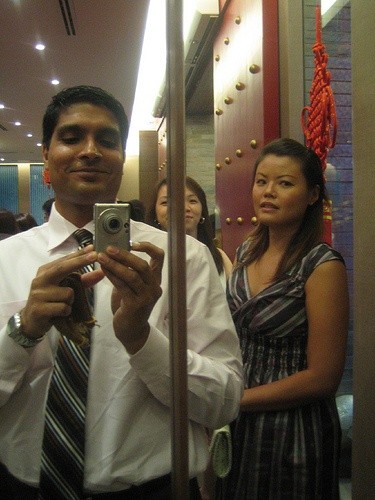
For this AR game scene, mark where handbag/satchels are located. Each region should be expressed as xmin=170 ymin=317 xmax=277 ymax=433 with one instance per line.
xmin=209 ymin=423 xmax=233 ymax=479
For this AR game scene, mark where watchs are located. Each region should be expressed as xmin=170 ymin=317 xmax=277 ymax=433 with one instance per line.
xmin=5 ymin=314 xmax=22 ymax=337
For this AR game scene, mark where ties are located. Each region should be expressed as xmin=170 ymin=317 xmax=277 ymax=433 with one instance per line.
xmin=38 ymin=228 xmax=94 ymax=499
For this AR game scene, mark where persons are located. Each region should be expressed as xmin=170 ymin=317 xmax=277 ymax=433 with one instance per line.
xmin=0 ymin=86 xmax=245 ymax=500
xmin=147 ymin=175 xmax=233 ymax=288
xmin=2 ymin=197 xmax=53 ymax=240
xmin=226 ymin=137 xmax=350 ymax=498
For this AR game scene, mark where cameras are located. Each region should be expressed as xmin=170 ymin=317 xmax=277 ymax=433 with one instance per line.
xmin=92 ymin=202 xmax=131 ymax=259
